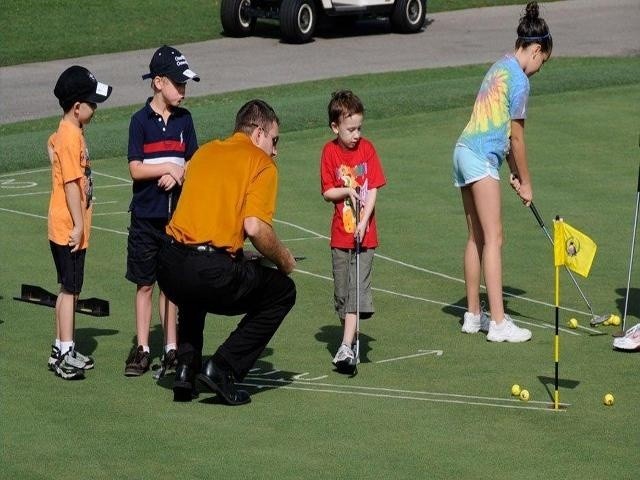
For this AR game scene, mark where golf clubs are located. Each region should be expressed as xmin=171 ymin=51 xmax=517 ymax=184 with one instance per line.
xmin=64 ymin=251 xmax=86 ymax=369
xmin=529 ymin=201 xmax=611 ymax=325
xmin=348 ymin=185 xmax=363 ymax=366
xmin=612 ymin=175 xmax=640 ymax=337
xmin=153 ymin=190 xmax=174 ymax=379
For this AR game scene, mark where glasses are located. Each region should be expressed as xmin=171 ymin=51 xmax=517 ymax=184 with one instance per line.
xmin=251 ymin=122 xmax=279 ymax=146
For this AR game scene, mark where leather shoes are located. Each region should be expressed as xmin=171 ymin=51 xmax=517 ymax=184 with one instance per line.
xmin=198 ymin=358 xmax=252 ymax=405
xmin=172 ymin=365 xmax=199 ymax=399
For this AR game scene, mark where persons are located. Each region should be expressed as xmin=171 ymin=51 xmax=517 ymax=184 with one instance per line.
xmin=453 ymin=0 xmax=553 ymax=344
xmin=46 ymin=66 xmax=114 ymax=379
xmin=157 ymin=99 xmax=297 ymax=406
xmin=124 ymin=43 xmax=200 ymax=375
xmin=320 ymin=89 xmax=387 ymax=374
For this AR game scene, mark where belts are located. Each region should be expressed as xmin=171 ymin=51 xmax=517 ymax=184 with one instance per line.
xmin=168 ymin=239 xmax=220 ymax=253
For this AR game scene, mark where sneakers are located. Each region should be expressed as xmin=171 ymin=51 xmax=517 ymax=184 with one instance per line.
xmin=461 ymin=311 xmax=513 ymax=334
xmin=164 ymin=349 xmax=178 ymax=376
xmin=47 ymin=345 xmax=95 ymax=380
xmin=124 ymin=348 xmax=151 ymax=377
xmin=332 ymin=344 xmax=360 ymax=374
xmin=486 ymin=320 xmax=532 ymax=343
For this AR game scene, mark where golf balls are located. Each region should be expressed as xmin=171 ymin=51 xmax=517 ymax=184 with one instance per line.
xmin=519 ymin=389 xmax=530 ymax=399
xmin=601 ymin=314 xmax=619 ymax=328
xmin=570 ymin=318 xmax=577 ymax=329
xmin=512 ymin=384 xmax=522 ymax=398
xmin=604 ymin=394 xmax=615 ymax=406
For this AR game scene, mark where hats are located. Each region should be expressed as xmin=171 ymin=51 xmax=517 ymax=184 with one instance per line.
xmin=54 ymin=65 xmax=112 ymax=103
xmin=149 ymin=45 xmax=200 ymax=83
